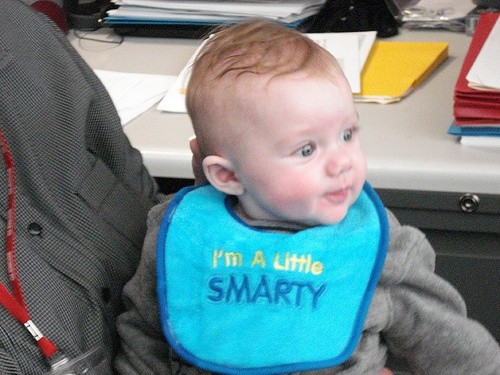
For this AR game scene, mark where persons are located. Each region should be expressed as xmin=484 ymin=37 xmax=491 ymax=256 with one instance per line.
xmin=0 ymin=0 xmax=203 ymax=375
xmin=110 ymin=16 xmax=500 ymax=375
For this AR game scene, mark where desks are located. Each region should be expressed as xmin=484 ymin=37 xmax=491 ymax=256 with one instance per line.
xmin=65 ymin=0 xmax=498 ymax=375
